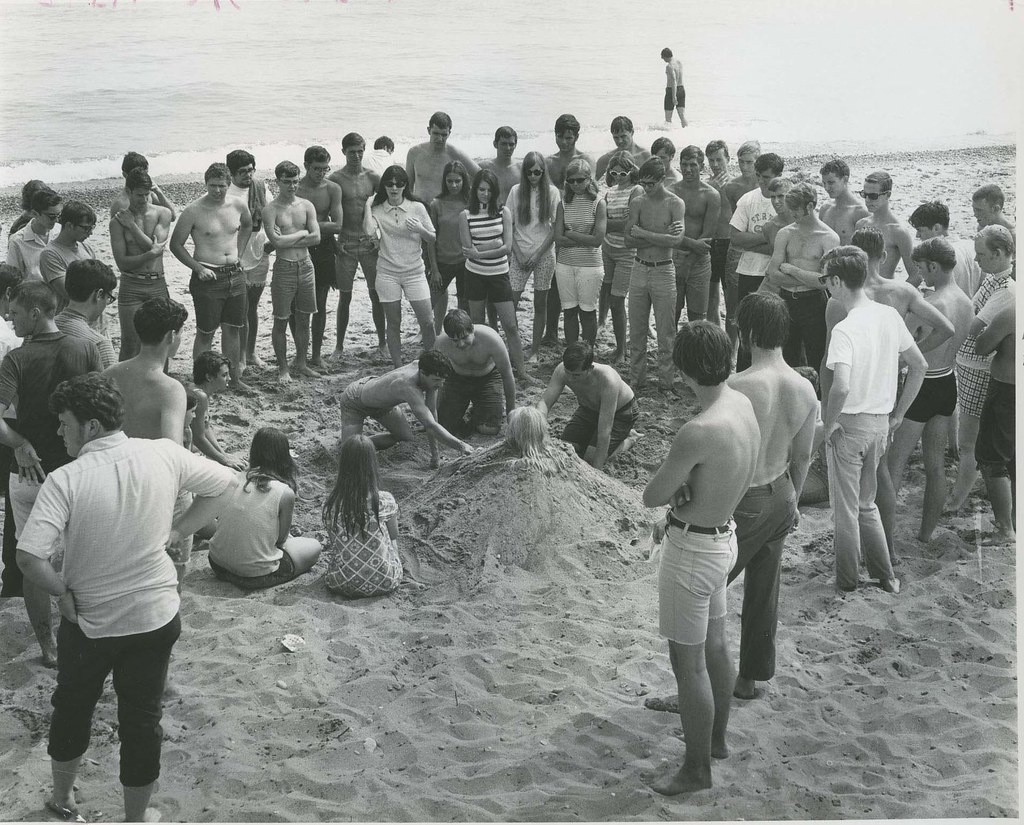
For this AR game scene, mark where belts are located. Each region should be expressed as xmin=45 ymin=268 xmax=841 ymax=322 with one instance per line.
xmin=121 ymin=271 xmax=164 ymax=280
xmin=201 ymin=261 xmax=241 ymax=274
xmin=615 ymin=395 xmax=636 ymax=417
xmin=635 ymin=256 xmax=672 ymax=267
xmin=665 ymin=507 xmax=732 ymax=534
xmin=779 ymin=288 xmax=823 ymax=300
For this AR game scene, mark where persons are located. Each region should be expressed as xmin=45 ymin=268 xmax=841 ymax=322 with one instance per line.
xmin=425 ymin=310 xmax=516 ymax=471
xmin=0 ymin=180 xmax=109 ymax=338
xmin=819 ymin=159 xmax=1016 ymax=593
xmin=111 ymin=151 xmax=176 ymax=222
xmin=0 ymin=262 xmax=24 ymax=598
xmin=208 ymin=427 xmax=320 ymax=589
xmin=661 ymin=47 xmax=688 ymax=128
xmin=170 ymin=112 xmax=840 ymax=400
xmin=0 ymin=281 xmax=104 ymax=670
xmin=643 ymin=320 xmax=760 ymax=795
xmin=101 ymin=298 xmax=244 ymax=663
xmin=110 ymin=168 xmax=172 ymax=374
xmin=787 ymin=366 xmax=830 ymax=506
xmin=16 ymin=371 xmax=239 ymax=822
xmin=644 ymin=290 xmax=818 ymax=714
xmin=322 ymin=434 xmax=403 ymax=597
xmin=536 ymin=341 xmax=643 ymax=472
xmin=506 ymin=406 xmax=565 ymax=472
xmin=54 ymin=258 xmax=118 ymax=369
xmin=340 ymin=350 xmax=474 ymax=470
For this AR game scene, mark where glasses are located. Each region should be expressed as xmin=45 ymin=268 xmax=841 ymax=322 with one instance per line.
xmin=95 ymin=288 xmax=116 ymax=306
xmin=384 ymin=180 xmax=406 ymax=188
xmin=566 ymin=176 xmax=587 ymax=184
xmin=860 ymin=190 xmax=888 ymax=200
xmin=610 ymin=169 xmax=631 ymax=179
xmin=70 ymin=222 xmax=96 ymax=230
xmin=526 ymin=169 xmax=544 ymax=176
xmin=232 ymin=167 xmax=256 ymax=177
xmin=37 ymin=210 xmax=61 ymax=220
xmin=818 ymin=274 xmax=841 ymax=285
xmin=308 ymin=163 xmax=330 ymax=175
